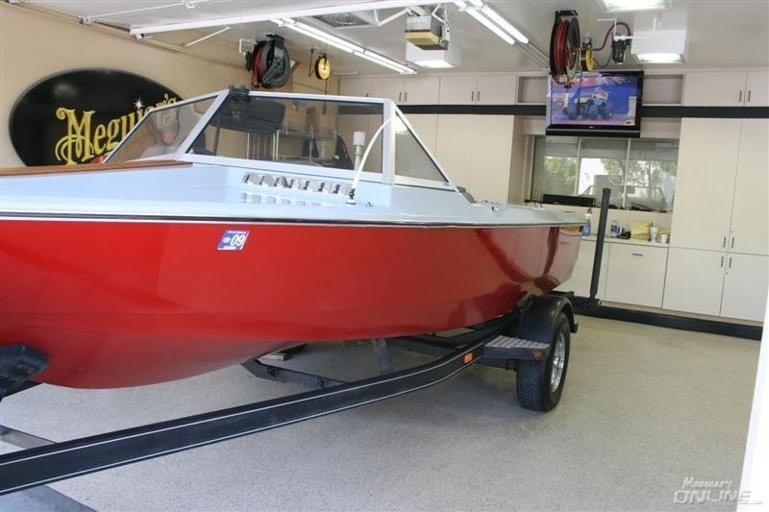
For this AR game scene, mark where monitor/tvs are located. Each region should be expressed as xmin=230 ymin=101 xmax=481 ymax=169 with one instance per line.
xmin=545 ymin=67 xmax=644 ymax=137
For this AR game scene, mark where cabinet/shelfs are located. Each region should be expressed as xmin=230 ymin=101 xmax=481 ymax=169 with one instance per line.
xmin=338 ymin=78 xmax=374 ymax=100
xmin=372 ymin=75 xmax=438 ymax=106
xmin=564 ymin=235 xmax=668 ymax=312
xmin=439 ymin=75 xmax=516 ymax=103
xmin=402 ymin=113 xmax=529 ymax=209
xmin=681 ymin=70 xmax=769 ymax=104
xmin=665 ymin=115 xmax=768 ymax=320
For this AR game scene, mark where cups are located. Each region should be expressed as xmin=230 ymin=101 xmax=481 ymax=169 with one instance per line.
xmin=649 ymin=226 xmax=658 ymax=244
xmin=661 ymin=234 xmax=667 ymax=243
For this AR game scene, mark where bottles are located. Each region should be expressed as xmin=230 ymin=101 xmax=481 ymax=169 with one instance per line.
xmin=610 ymin=218 xmax=619 ymax=238
xmin=583 ymin=219 xmax=591 ymax=236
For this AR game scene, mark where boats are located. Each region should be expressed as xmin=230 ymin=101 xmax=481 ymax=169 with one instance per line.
xmin=0 ymin=80 xmax=590 ymax=395
